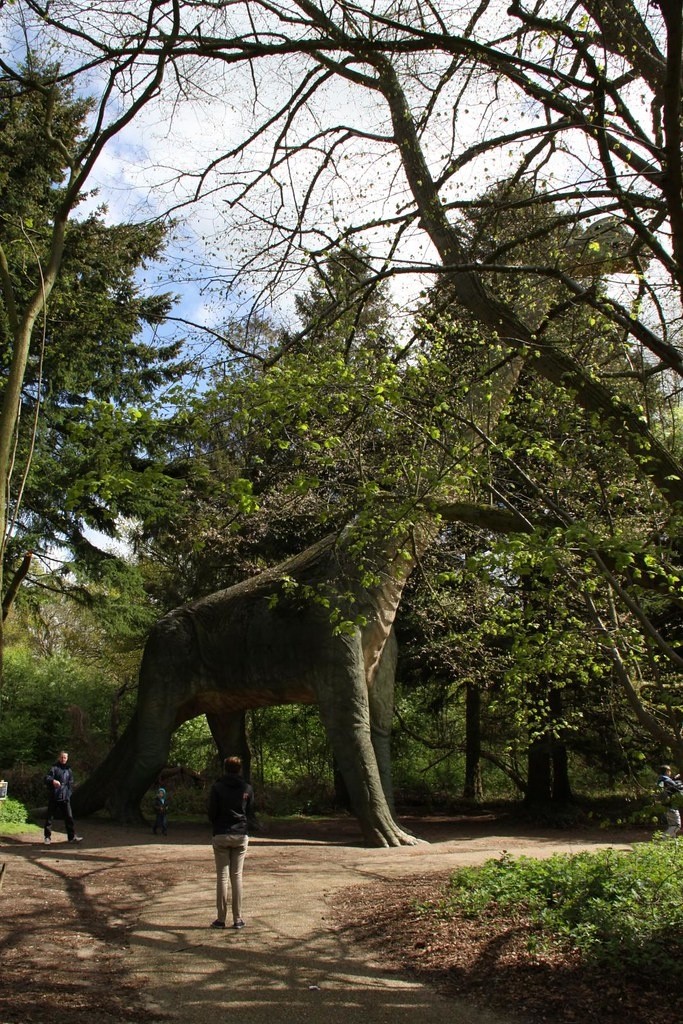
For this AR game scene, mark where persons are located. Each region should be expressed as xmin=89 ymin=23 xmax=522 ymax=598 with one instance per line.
xmin=152 ymin=788 xmax=169 ymax=836
xmin=44 ymin=751 xmax=83 ymax=844
xmin=208 ymin=757 xmax=255 ymax=929
xmin=657 ymin=766 xmax=683 ymax=840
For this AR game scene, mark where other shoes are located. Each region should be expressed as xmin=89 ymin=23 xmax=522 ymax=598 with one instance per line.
xmin=162 ymin=831 xmax=168 ymax=837
xmin=153 ymin=830 xmax=157 ymax=835
xmin=211 ymin=919 xmax=225 ymax=929
xmin=44 ymin=837 xmax=51 ymax=845
xmin=68 ymin=836 xmax=83 ymax=844
xmin=233 ymin=920 xmax=245 ymax=930
